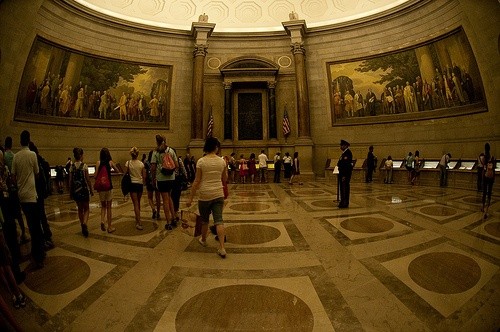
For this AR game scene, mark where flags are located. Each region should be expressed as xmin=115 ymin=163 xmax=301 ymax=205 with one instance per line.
xmin=282 ymin=105 xmax=291 ymax=137
xmin=203 ymin=107 xmax=213 ymax=141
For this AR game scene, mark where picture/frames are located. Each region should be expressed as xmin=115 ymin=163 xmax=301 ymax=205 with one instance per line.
xmin=325 ymin=24 xmax=489 ymax=127
xmin=14 ymin=35 xmax=174 ymax=130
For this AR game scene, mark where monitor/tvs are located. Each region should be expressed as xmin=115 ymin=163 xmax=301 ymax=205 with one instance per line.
xmin=87 ymin=166 xmax=96 ymax=175
xmin=50 ymin=168 xmax=57 ymax=176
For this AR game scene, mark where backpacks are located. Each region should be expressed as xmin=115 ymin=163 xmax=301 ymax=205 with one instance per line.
xmin=94 ymin=165 xmax=110 ymax=190
xmin=70 ymin=164 xmax=87 ymax=198
xmin=160 ymin=146 xmax=175 ymax=175
xmin=386 ymin=161 xmax=391 ymax=169
xmin=484 ymin=158 xmax=495 ymax=178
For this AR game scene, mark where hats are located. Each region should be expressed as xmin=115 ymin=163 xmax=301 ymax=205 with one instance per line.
xmin=340 ymin=139 xmax=350 ymax=146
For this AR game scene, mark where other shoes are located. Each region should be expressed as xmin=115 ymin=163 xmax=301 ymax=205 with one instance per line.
xmin=107 ymin=227 xmax=115 ymax=233
xmin=14 ymin=251 xmax=31 ymax=260
xmin=136 ymin=224 xmax=143 ymax=230
xmin=158 ymin=214 xmax=161 ymax=217
xmin=20 ymin=235 xmax=30 ymax=240
xmin=44 ymin=240 xmax=54 ymax=250
xmin=164 ymin=224 xmax=172 ymax=230
xmin=152 ymin=210 xmax=157 ymax=219
xmin=171 ymin=219 xmax=176 ymax=227
xmin=215 ymin=235 xmax=226 ymax=242
xmin=82 ymin=223 xmax=89 ymax=237
xmin=411 ymin=181 xmax=414 ymax=184
xmin=484 ymin=214 xmax=488 ymax=218
xmin=217 ymin=248 xmax=226 ymax=257
xmin=101 ymin=222 xmax=106 ymax=231
xmin=232 ymin=180 xmax=303 ymax=185
xmin=199 ymin=237 xmax=207 ymax=246
xmin=338 ymin=205 xmax=350 ymax=208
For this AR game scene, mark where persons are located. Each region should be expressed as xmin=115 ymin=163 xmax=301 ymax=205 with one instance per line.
xmin=222 ymin=150 xmax=305 ymax=184
xmin=337 ymin=138 xmax=353 ymax=209
xmin=69 ymin=147 xmax=93 ymax=238
xmin=142 ymin=135 xmax=197 ymax=229
xmin=365 ymin=145 xmax=452 ymax=186
xmin=0 ymin=130 xmax=74 ymax=309
xmin=93 ymin=148 xmax=119 ymax=233
xmin=334 ymin=63 xmax=477 ymax=120
xmin=123 ymin=146 xmax=146 ymax=230
xmin=205 ymin=150 xmax=229 ymax=242
xmin=187 ymin=137 xmax=229 ymax=256
xmin=26 ymin=72 xmax=166 ymax=122
xmin=475 ymin=143 xmax=497 ymax=218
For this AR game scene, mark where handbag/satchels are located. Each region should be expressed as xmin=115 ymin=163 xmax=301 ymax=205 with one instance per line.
xmin=181 ymin=204 xmax=201 ymax=235
xmin=243 ymin=164 xmax=249 ymax=170
xmin=178 ymin=174 xmax=188 ymax=191
xmin=229 ymin=164 xmax=234 ymax=170
xmin=121 ymin=162 xmax=132 ymax=196
